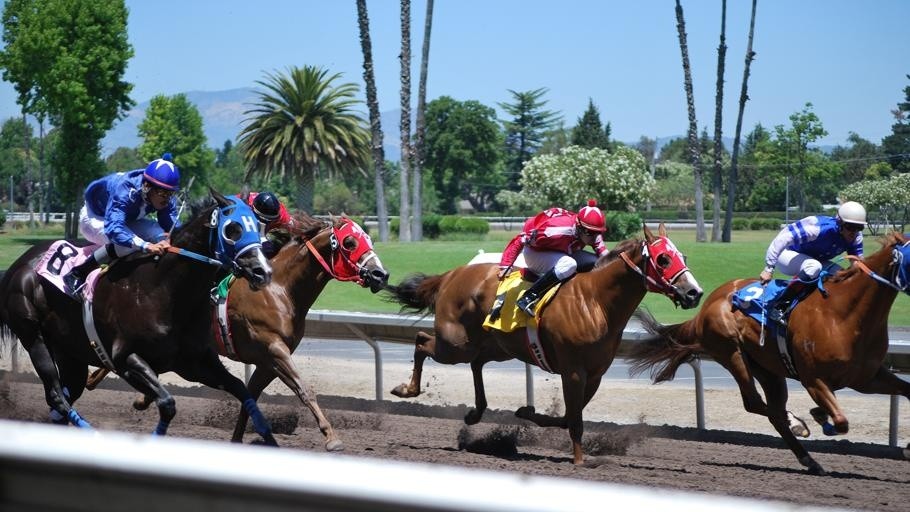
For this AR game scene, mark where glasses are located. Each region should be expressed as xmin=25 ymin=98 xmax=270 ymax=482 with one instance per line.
xmin=584 ymin=232 xmax=602 ymax=238
xmin=157 ymin=190 xmax=174 ymax=199
xmin=844 ymin=223 xmax=864 ymax=232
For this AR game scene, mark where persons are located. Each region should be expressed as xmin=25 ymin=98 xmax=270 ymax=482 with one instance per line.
xmin=209 ymin=190 xmax=305 ymax=307
xmin=757 ymin=199 xmax=870 ymax=328
xmin=59 ymin=157 xmax=186 ymax=304
xmin=495 ymin=204 xmax=610 ymax=319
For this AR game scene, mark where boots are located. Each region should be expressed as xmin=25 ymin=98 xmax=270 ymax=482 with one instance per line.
xmin=768 ymin=270 xmax=813 ymax=326
xmin=516 ymin=267 xmax=559 ymax=318
xmin=63 ymin=243 xmax=118 ymax=303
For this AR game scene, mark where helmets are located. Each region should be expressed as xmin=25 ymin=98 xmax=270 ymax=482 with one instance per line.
xmin=252 ymin=192 xmax=282 ymax=221
xmin=142 ymin=152 xmax=182 ymax=191
xmin=836 ymin=200 xmax=868 ymax=225
xmin=577 ymin=200 xmax=607 ymax=232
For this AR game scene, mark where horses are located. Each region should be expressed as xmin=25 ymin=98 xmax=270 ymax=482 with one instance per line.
xmin=132 ymin=209 xmax=391 ymax=452
xmin=375 ymin=220 xmax=705 ymax=467
xmin=620 ymin=229 xmax=910 ymax=476
xmin=0 ymin=181 xmax=280 ymax=448
